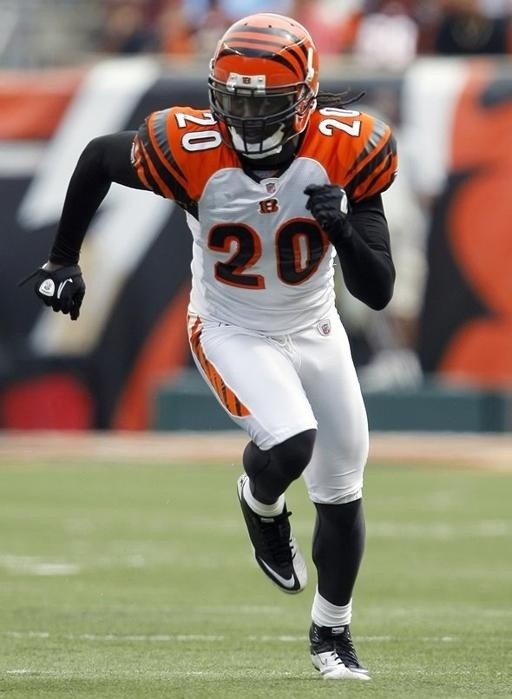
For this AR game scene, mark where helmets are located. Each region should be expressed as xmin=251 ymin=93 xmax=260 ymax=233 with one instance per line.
xmin=208 ymin=12 xmax=320 ymax=166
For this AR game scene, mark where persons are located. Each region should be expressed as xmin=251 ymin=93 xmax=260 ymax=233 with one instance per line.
xmin=31 ymin=11 xmax=398 ymax=684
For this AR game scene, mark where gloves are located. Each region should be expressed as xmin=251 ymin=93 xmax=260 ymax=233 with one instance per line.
xmin=303 ymin=182 xmax=350 ymax=231
xmin=32 ymin=263 xmax=86 ymax=319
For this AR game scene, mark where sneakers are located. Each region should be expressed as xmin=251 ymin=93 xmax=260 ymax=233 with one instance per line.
xmin=309 ymin=622 xmax=372 ymax=682
xmin=236 ymin=472 xmax=310 ymax=594
